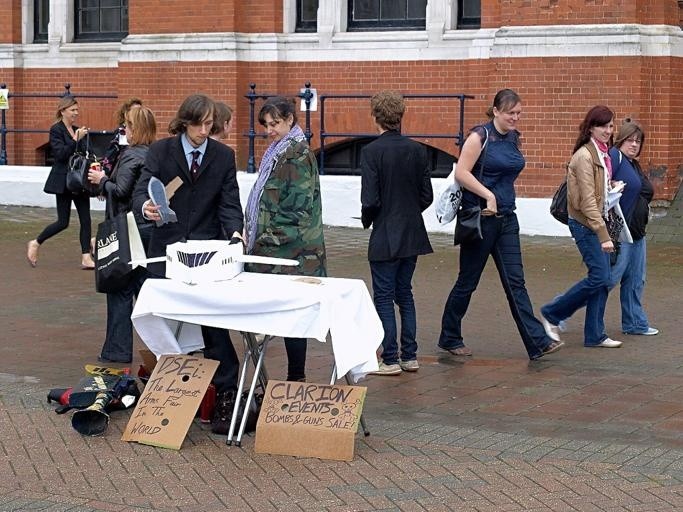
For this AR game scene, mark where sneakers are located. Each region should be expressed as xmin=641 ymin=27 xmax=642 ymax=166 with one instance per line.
xmin=540 ymin=306 xmax=561 ymax=342
xmin=367 ymin=359 xmax=402 ymax=376
xmin=623 ymin=327 xmax=659 ymax=336
xmin=401 ymin=357 xmax=420 ymax=371
xmin=595 ymin=336 xmax=622 ymax=349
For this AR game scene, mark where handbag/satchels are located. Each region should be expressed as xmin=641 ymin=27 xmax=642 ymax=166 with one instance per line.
xmin=94 ymin=179 xmax=148 ymax=293
xmin=65 ymin=125 xmax=101 ymax=200
xmin=451 ymin=125 xmax=483 ymax=248
xmin=437 ymin=161 xmax=463 ymax=226
xmin=549 ymin=160 xmax=571 ymax=227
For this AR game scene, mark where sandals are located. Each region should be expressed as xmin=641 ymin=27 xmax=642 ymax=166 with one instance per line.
xmin=26 ymin=240 xmax=38 ymax=267
xmin=80 ymin=259 xmax=96 ymax=270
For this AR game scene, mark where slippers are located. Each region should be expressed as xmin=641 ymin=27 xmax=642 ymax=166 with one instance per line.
xmin=437 ymin=342 xmax=473 ymax=357
xmin=529 ymin=341 xmax=565 ymax=362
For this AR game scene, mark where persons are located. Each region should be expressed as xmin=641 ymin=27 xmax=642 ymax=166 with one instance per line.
xmin=549 ymin=122 xmax=659 ymax=336
xmin=434 ymin=88 xmax=564 ymax=361
xmin=25 ymin=92 xmax=99 ymax=270
xmin=132 ymin=94 xmax=244 ymax=388
xmin=245 ymin=96 xmax=327 ymax=387
xmin=538 ymin=104 xmax=633 ymax=349
xmin=88 ymin=107 xmax=157 ymax=363
xmin=209 ymin=102 xmax=233 ymax=142
xmin=101 ymin=97 xmax=143 ymax=177
xmin=360 ymin=89 xmax=434 ymax=376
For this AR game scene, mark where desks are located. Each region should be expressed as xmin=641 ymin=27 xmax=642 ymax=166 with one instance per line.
xmin=130 ymin=275 xmax=386 ymax=448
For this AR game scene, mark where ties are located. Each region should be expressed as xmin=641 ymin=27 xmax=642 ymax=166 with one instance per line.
xmin=189 ymin=150 xmax=201 ymax=184
